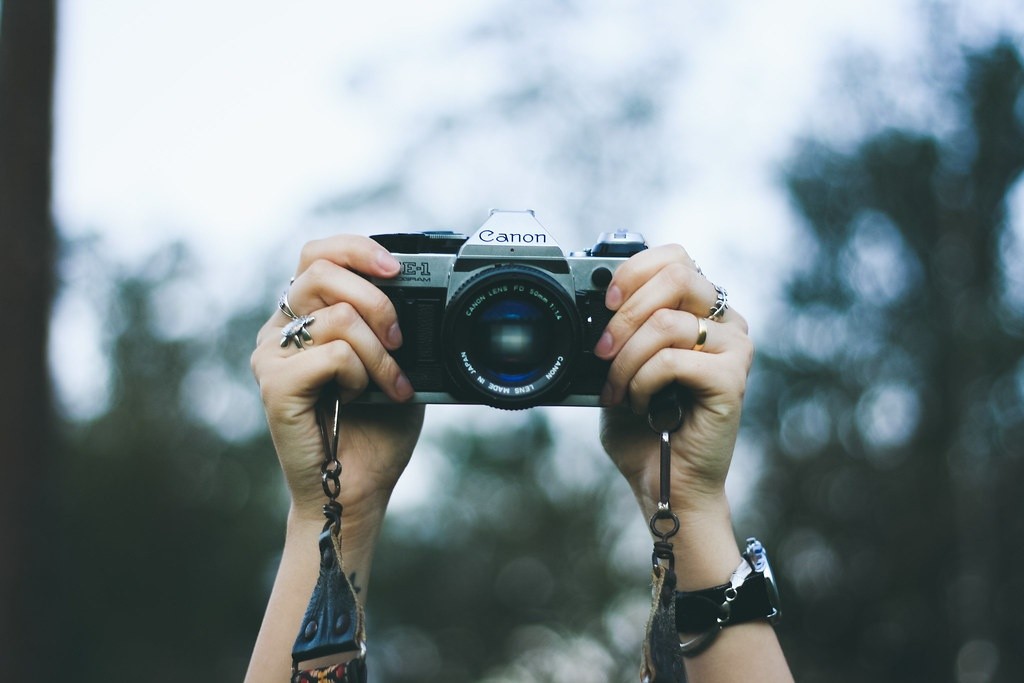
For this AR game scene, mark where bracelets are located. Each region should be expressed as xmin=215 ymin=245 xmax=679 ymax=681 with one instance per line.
xmin=678 ymin=557 xmax=755 ymax=657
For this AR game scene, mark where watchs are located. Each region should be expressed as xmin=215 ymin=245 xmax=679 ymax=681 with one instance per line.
xmin=676 ymin=536 xmax=784 ymax=631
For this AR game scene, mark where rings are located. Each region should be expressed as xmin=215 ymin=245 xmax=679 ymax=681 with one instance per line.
xmin=707 ymin=282 xmax=731 ymax=321
xmin=277 ymin=288 xmax=298 ymax=320
xmin=692 ymin=313 xmax=706 ymax=351
xmin=693 ymin=259 xmax=707 ymax=277
xmin=288 ymin=275 xmax=296 ymax=285
xmin=280 ymin=312 xmax=315 ymax=351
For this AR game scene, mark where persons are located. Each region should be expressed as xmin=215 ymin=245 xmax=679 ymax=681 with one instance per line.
xmin=245 ymin=235 xmax=795 ymax=683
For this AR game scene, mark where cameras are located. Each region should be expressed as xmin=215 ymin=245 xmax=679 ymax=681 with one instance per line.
xmin=330 ymin=208 xmax=649 ymax=409
